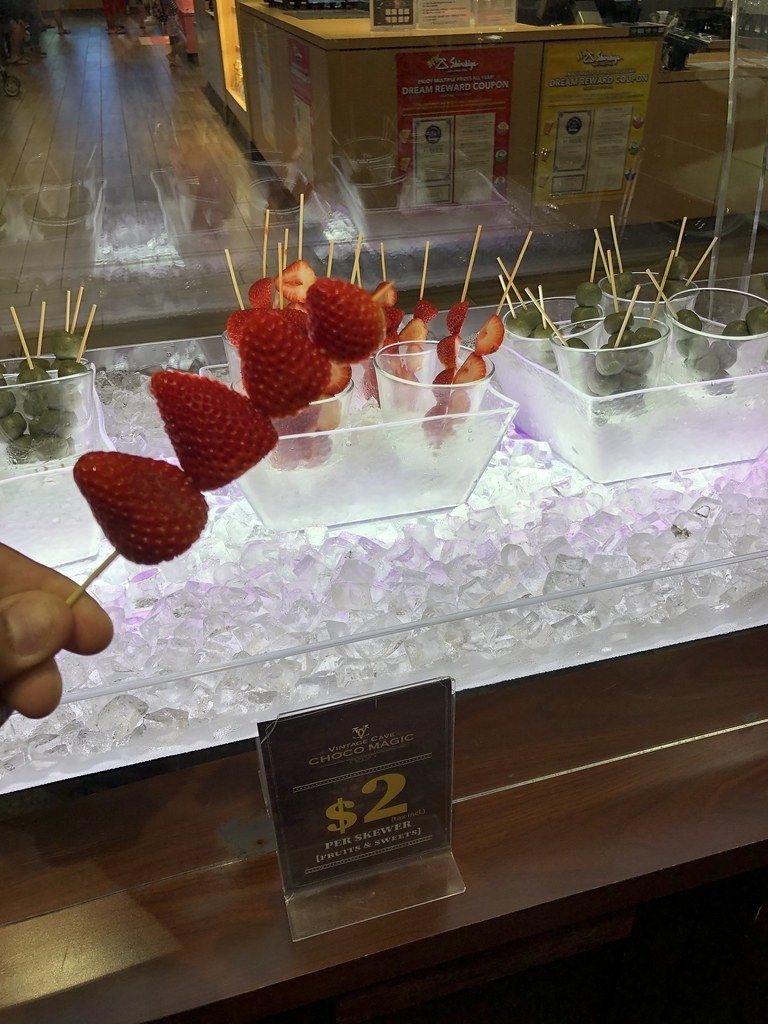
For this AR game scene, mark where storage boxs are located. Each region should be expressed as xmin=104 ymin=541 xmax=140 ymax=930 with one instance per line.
xmin=197 ymin=363 xmax=521 ymax=532
xmin=1 ymin=390 xmax=122 ymax=569
xmin=493 ymin=332 xmax=768 ymax=485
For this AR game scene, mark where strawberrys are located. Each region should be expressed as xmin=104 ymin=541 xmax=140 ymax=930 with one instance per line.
xmin=71 ymin=258 xmax=505 ymax=564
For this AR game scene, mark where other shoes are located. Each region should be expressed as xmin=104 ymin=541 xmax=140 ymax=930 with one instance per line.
xmin=58 ymin=29 xmax=71 ymax=34
xmin=106 ymin=25 xmax=126 ymax=34
xmin=43 ymin=23 xmax=55 ymax=28
xmin=166 ymin=54 xmax=179 ymax=67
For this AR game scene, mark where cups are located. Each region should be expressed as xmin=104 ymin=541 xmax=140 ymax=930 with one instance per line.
xmin=501 ymin=271 xmax=768 ymax=399
xmin=222 ymin=320 xmax=497 ymax=473
xmin=0 ymin=356 xmax=96 ymax=472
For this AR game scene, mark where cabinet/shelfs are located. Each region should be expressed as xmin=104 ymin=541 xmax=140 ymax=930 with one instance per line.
xmin=192 ymin=0 xmax=663 ymax=236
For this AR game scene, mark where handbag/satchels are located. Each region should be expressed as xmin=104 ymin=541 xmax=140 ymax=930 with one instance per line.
xmin=165 ymin=13 xmax=184 ymax=37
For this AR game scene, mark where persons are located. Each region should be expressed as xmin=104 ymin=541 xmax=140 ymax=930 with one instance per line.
xmin=0 ymin=0 xmax=208 ymax=72
xmin=0 ymin=547 xmax=117 ymax=741
